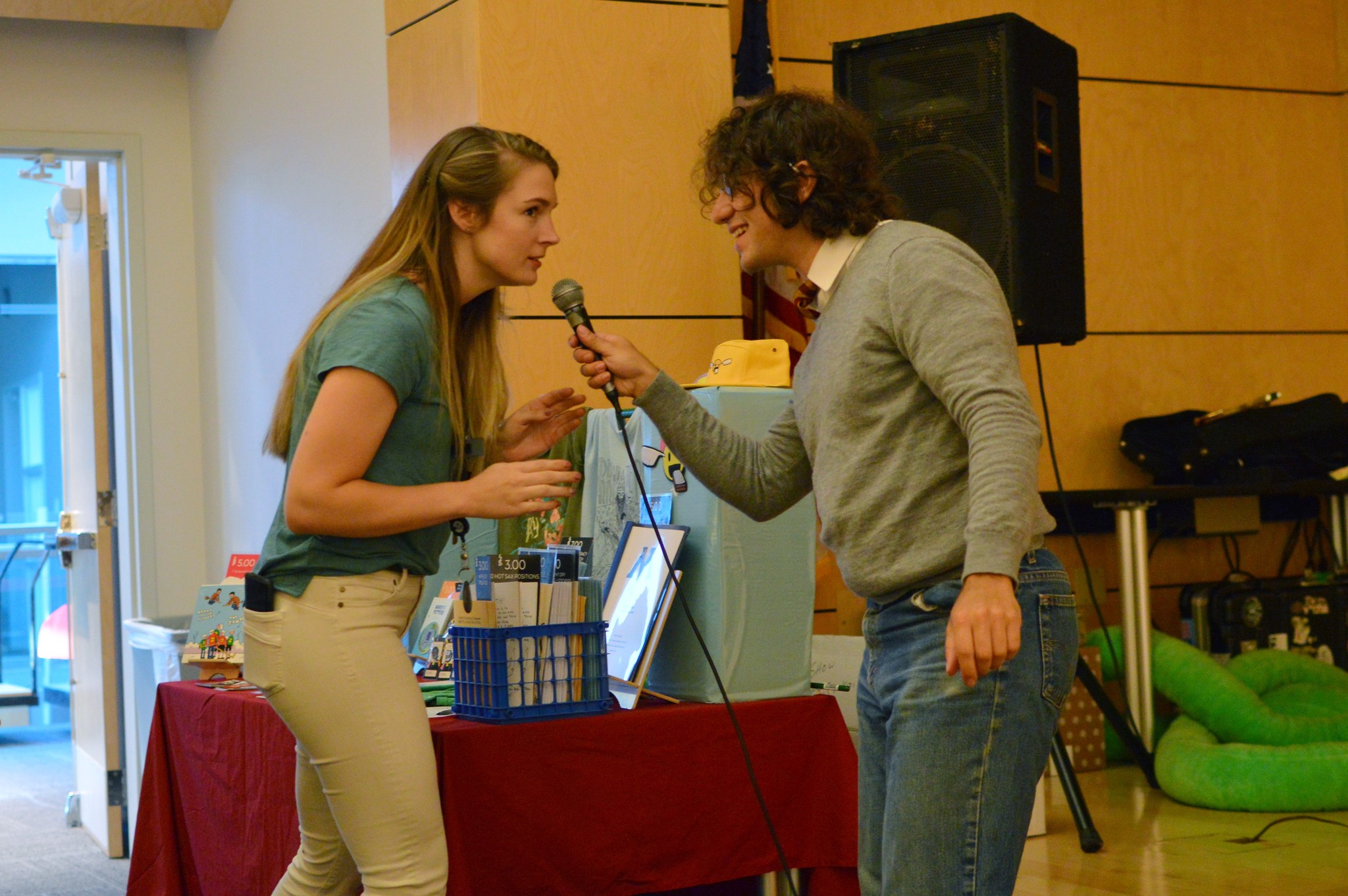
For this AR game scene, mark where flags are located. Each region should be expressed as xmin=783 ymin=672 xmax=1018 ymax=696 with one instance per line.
xmin=733 ymin=0 xmax=822 ymax=376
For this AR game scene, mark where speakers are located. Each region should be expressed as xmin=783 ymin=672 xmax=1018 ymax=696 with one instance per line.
xmin=831 ymin=13 xmax=1088 ymax=348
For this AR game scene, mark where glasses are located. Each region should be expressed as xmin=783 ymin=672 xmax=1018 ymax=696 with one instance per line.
xmin=716 ymin=169 xmax=767 ymax=197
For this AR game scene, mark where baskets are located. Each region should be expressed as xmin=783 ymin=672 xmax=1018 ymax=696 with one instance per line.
xmin=447 ymin=621 xmax=613 ymax=724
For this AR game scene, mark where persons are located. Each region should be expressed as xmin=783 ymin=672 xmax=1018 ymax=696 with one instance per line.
xmin=240 ymin=125 xmax=588 ymax=895
xmin=568 ymin=87 xmax=1081 ymax=896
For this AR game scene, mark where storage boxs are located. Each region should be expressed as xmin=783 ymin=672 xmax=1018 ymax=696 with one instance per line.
xmin=448 ymin=620 xmax=614 ymax=725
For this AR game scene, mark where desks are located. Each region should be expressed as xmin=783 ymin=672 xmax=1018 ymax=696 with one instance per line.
xmin=125 ymin=674 xmax=863 ymax=895
xmin=1037 ymin=486 xmax=1348 ymax=758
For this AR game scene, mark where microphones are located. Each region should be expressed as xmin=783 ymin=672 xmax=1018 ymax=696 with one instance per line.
xmin=550 ymin=278 xmax=623 ymax=414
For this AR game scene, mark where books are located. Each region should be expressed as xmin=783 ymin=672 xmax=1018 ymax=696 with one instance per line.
xmin=410 ymin=580 xmax=463 ymax=657
xmin=181 ymin=554 xmax=261 ymax=668
